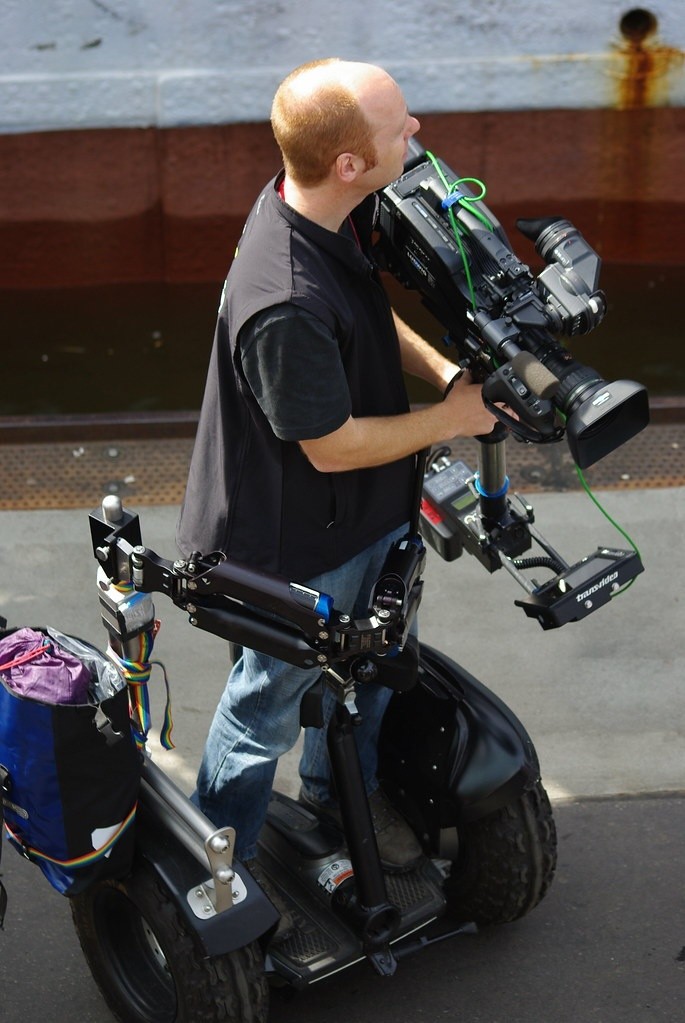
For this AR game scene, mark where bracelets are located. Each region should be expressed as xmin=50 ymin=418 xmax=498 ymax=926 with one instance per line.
xmin=443 ymin=367 xmax=469 ymax=399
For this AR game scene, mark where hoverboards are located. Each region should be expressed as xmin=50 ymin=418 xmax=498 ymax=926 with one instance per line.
xmin=1 ymin=481 xmax=563 ymax=1023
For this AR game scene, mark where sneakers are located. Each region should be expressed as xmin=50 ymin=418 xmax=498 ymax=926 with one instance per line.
xmin=298 ymin=779 xmax=426 ymax=876
xmin=237 ymin=854 xmax=297 ymax=946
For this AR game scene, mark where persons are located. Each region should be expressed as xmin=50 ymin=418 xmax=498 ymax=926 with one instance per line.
xmin=176 ymin=58 xmax=519 ymax=944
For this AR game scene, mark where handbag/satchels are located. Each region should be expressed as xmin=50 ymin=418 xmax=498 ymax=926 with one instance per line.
xmin=0 ymin=627 xmax=145 ymax=896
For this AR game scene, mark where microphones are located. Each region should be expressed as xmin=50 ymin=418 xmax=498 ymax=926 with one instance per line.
xmin=473 ymin=310 xmax=560 ymax=401
